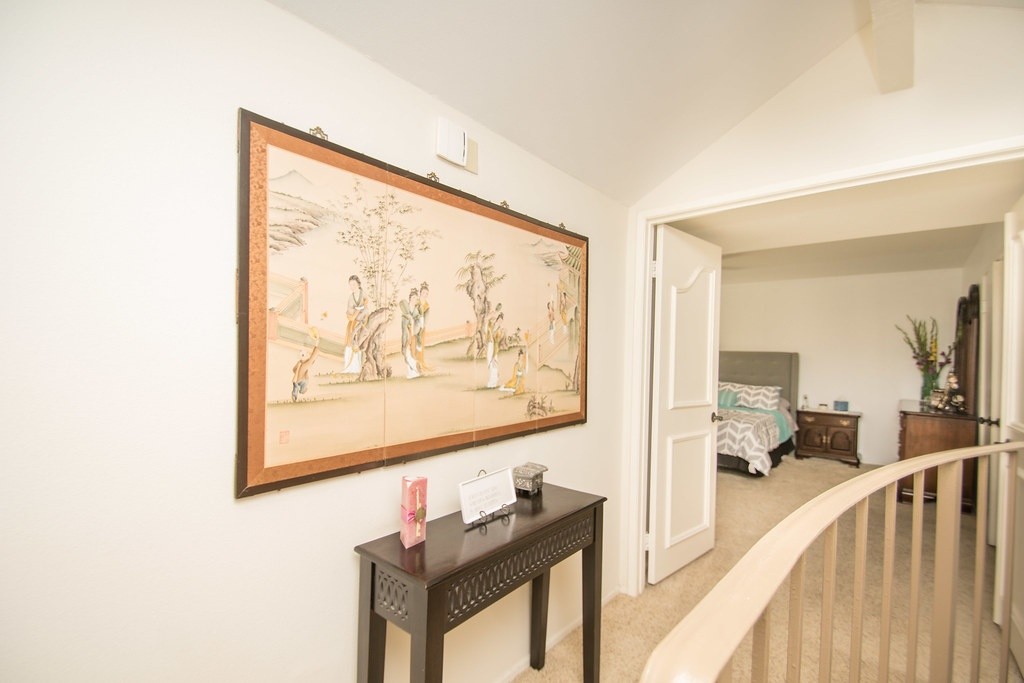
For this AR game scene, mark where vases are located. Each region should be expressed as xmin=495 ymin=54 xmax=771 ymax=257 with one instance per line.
xmin=922 ymin=374 xmax=939 ymax=398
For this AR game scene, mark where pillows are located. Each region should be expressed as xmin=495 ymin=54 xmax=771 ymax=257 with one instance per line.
xmin=718 ymin=381 xmax=790 ymax=411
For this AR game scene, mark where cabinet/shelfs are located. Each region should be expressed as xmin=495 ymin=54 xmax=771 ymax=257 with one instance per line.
xmin=897 ymin=399 xmax=978 ymax=513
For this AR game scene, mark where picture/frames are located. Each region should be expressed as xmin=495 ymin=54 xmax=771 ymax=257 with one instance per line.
xmin=236 ymin=108 xmax=589 ymax=501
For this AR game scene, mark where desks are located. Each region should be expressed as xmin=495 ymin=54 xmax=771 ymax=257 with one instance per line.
xmin=354 ymin=482 xmax=608 ymax=683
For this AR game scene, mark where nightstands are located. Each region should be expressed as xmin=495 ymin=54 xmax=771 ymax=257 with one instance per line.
xmin=796 ymin=410 xmax=863 ymax=468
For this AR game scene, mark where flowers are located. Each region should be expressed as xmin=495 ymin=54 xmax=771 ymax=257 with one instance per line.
xmin=895 ymin=314 xmax=958 ymax=380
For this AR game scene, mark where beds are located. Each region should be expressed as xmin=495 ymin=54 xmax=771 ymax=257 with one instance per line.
xmin=717 ymin=350 xmax=799 ymax=477
